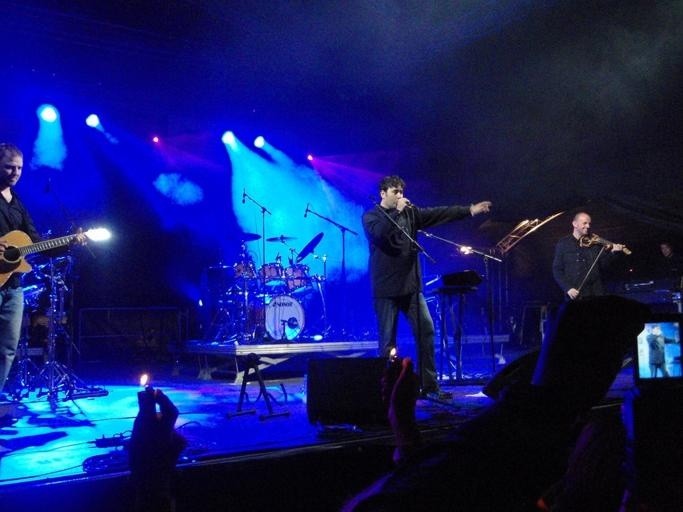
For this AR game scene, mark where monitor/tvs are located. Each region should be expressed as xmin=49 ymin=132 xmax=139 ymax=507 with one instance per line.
xmin=633 ymin=312 xmax=683 ymax=389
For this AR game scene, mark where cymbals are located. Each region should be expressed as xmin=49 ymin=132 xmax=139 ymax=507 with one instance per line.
xmin=267 ymin=237 xmax=292 ymax=241
xmin=226 ymin=233 xmax=261 ymax=241
xmin=209 ymin=266 xmax=231 ymax=270
xmin=296 ymin=232 xmax=324 ymax=262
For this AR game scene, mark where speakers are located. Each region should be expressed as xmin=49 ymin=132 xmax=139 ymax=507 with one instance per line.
xmin=307 ymin=357 xmax=404 ymax=425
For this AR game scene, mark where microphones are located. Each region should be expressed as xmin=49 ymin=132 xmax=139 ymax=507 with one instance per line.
xmin=242 ymin=187 xmax=245 ymax=204
xmin=406 ymin=202 xmax=413 ymax=209
xmin=369 ymin=195 xmax=377 ymax=206
xmin=304 ymin=203 xmax=309 ymax=218
xmin=43 ymin=177 xmax=52 ymax=192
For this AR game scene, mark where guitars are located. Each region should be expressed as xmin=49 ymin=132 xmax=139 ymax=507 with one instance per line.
xmin=0 ymin=228 xmax=112 ymax=287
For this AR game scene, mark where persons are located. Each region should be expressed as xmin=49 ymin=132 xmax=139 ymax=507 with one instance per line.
xmin=550 ymin=212 xmax=626 ymax=303
xmin=359 ymin=174 xmax=493 ymax=400
xmin=342 ymin=294 xmax=656 ymax=512
xmin=0 ymin=141 xmax=90 ymax=394
xmin=657 ymin=239 xmax=682 ymax=277
xmin=377 ymin=354 xmax=425 ymax=467
xmin=125 ymin=384 xmax=189 ymax=511
xmin=644 ymin=322 xmax=681 ymax=379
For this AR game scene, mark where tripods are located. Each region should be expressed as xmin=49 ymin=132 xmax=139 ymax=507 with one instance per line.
xmin=204 ymin=213 xmax=358 ymax=342
xmin=375 ymin=207 xmax=462 ymax=412
xmin=11 ymin=255 xmax=89 ymax=414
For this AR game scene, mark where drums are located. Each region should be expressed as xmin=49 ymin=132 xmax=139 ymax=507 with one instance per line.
xmin=232 ymin=263 xmax=256 ymax=281
xmin=258 ymin=262 xmax=286 ymax=286
xmin=256 ymin=295 xmax=304 ymax=339
xmin=284 ymin=265 xmax=313 ymax=295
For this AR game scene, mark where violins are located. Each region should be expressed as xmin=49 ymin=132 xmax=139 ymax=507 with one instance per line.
xmin=580 ymin=232 xmax=631 ymax=256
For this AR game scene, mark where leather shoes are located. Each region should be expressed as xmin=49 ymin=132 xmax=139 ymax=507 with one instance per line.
xmin=422 ymin=388 xmax=452 ymax=398
